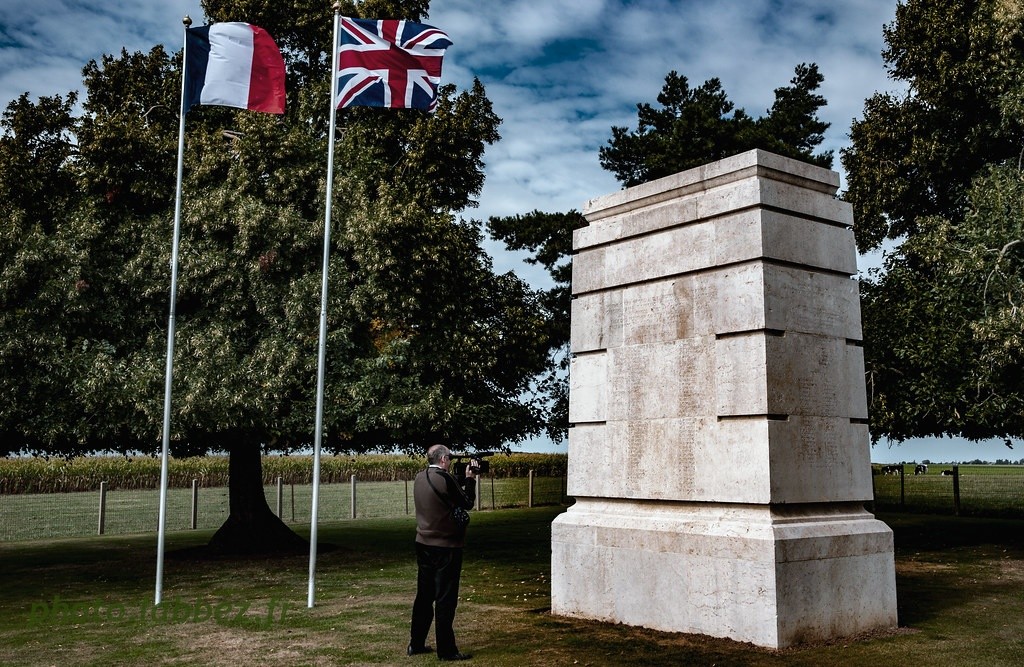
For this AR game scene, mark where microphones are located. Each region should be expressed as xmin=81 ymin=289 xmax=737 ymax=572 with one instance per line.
xmin=474 ymin=452 xmax=494 ymax=458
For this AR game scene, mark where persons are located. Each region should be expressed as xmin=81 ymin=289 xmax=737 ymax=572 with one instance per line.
xmin=407 ymin=445 xmax=481 ymax=661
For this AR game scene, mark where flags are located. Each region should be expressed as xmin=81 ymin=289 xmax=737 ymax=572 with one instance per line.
xmin=183 ymin=22 xmax=285 ymax=116
xmin=334 ymin=15 xmax=454 ymax=110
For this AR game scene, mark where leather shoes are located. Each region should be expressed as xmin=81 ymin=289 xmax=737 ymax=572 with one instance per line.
xmin=408 ymin=645 xmax=434 ymax=655
xmin=439 ymin=653 xmax=472 ymax=661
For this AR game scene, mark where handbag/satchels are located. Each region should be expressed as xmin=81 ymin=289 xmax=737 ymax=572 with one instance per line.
xmin=446 ymin=504 xmax=470 ymax=528
